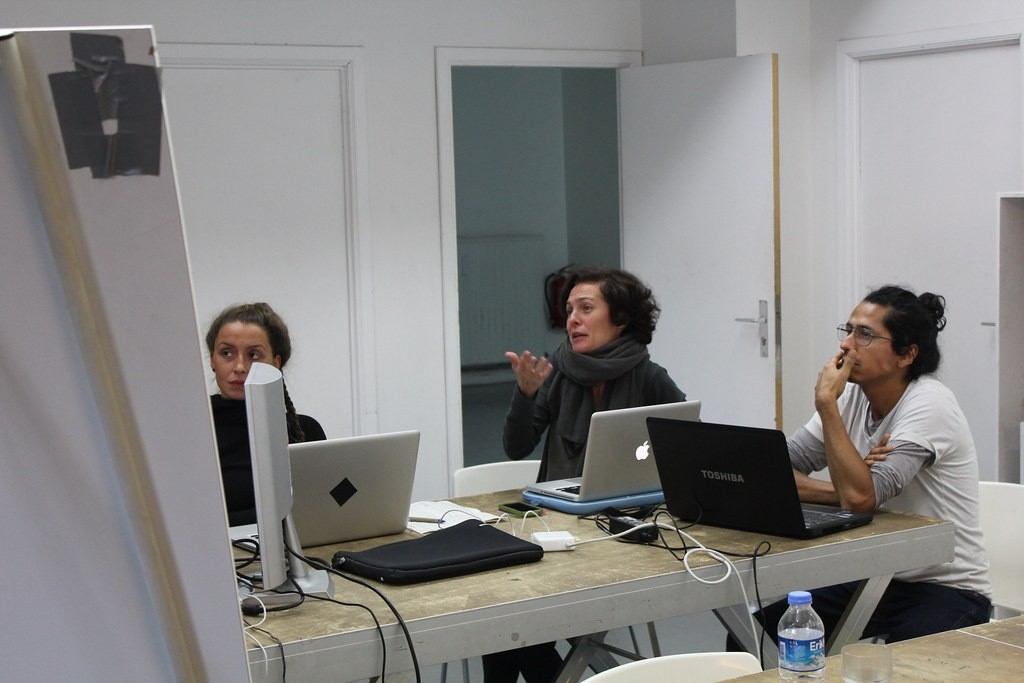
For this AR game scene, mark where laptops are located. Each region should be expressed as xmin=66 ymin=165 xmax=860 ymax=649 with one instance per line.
xmin=527 ymin=401 xmax=703 ymax=504
xmin=231 ymin=430 xmax=421 ymax=551
xmin=645 ymin=417 xmax=873 ymax=540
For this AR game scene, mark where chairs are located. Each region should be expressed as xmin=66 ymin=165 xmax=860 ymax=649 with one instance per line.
xmin=439 ymin=460 xmax=661 ymax=683
xmin=579 ymin=652 xmax=762 ymax=683
xmin=979 ymin=479 xmax=1023 ymax=623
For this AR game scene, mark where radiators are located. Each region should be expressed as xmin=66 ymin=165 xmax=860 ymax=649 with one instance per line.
xmin=455 ymin=232 xmax=549 ymax=373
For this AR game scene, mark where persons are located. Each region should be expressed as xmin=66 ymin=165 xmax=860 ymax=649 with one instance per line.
xmin=205 ymin=302 xmax=326 ymax=528
xmin=482 ymin=267 xmax=687 ymax=683
xmin=726 ymin=286 xmax=994 ymax=652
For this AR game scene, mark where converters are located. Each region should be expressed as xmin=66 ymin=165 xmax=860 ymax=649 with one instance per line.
xmin=610 ymin=517 xmax=659 ymax=544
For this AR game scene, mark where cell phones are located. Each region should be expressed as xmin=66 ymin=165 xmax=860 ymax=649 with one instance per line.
xmin=498 ymin=502 xmax=543 ymax=517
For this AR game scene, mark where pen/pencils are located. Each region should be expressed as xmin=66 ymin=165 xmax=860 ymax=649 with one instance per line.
xmin=409 ymin=516 xmax=446 ymax=524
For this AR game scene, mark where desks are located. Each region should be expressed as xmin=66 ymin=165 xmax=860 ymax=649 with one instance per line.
xmin=717 ymin=612 xmax=1023 ymax=683
xmin=230 ymin=488 xmax=955 ymax=683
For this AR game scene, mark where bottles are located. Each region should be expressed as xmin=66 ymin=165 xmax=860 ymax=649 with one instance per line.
xmin=777 ymin=589 xmax=826 ymax=683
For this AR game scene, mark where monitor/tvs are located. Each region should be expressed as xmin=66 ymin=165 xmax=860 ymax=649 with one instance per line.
xmin=236 ymin=361 xmax=335 ymax=603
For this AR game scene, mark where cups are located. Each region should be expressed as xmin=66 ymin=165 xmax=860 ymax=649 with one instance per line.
xmin=840 ymin=643 xmax=893 ymax=683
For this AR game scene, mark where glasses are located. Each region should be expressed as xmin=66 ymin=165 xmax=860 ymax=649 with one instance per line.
xmin=836 ymin=322 xmax=894 ymax=348
